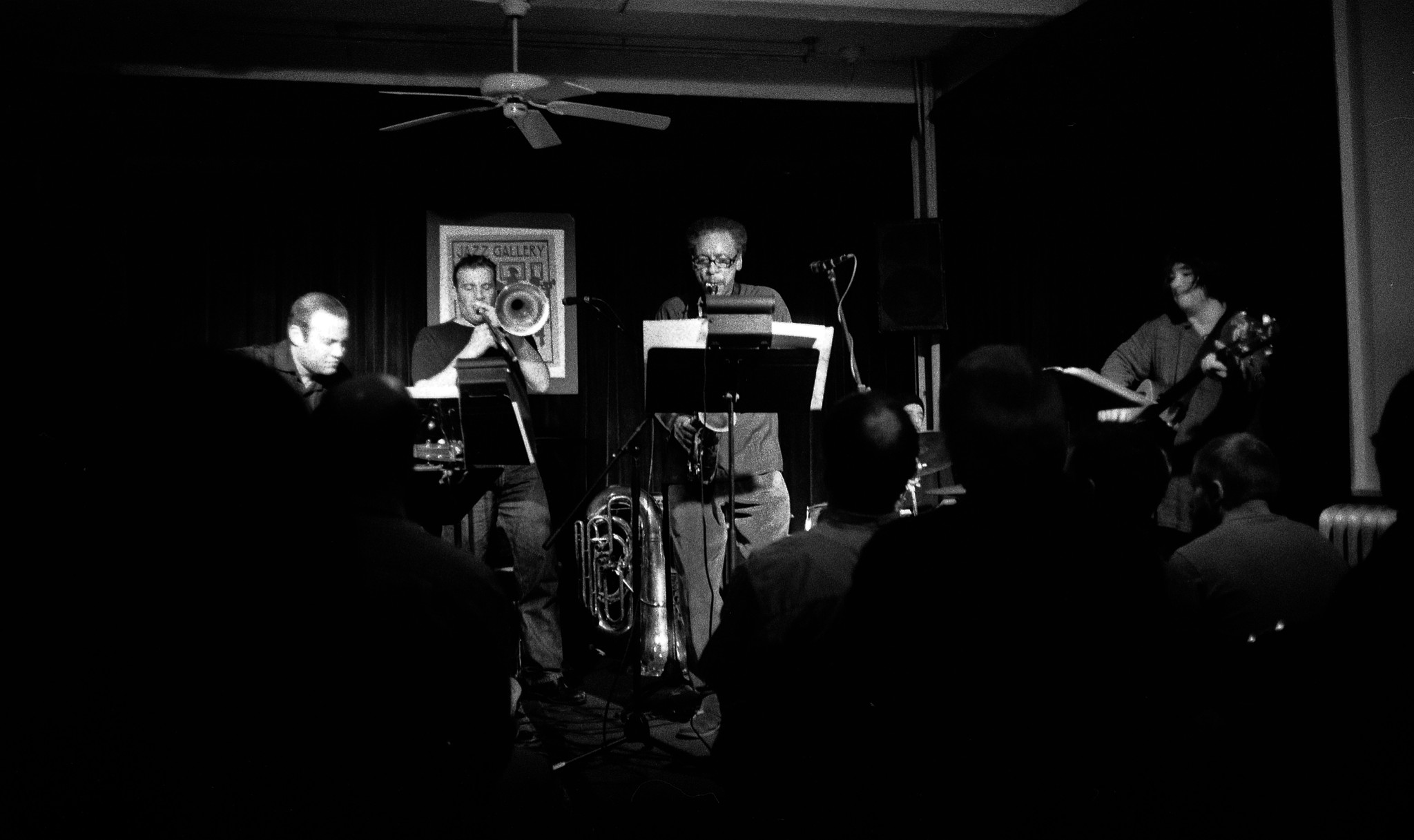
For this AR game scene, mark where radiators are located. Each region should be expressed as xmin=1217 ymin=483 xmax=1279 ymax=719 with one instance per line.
xmin=1321 ymin=504 xmax=1397 ymax=566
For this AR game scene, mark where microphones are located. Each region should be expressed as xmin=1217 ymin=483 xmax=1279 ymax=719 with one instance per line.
xmin=809 ymin=253 xmax=854 ymax=275
xmin=562 ymin=296 xmax=596 ymax=306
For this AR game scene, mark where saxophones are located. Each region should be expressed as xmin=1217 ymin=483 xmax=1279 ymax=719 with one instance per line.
xmin=681 ymin=284 xmax=741 ymax=487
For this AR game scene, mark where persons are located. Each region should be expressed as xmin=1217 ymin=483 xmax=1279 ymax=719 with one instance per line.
xmin=234 ymin=293 xmax=348 ymax=410
xmin=0 ymin=345 xmax=1414 ymax=840
xmin=411 ymin=255 xmax=586 ymax=705
xmin=1100 ymin=256 xmax=1268 ymax=549
xmin=651 ymin=218 xmax=793 ymax=740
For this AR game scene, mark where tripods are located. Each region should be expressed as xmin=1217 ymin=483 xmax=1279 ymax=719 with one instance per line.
xmin=546 ymin=310 xmax=714 ymax=775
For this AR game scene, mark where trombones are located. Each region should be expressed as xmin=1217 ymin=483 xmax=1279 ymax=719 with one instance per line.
xmin=466 ymin=279 xmax=553 ymax=365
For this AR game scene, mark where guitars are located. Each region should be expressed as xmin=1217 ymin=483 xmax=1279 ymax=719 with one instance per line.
xmin=1133 ymin=314 xmax=1278 ymax=427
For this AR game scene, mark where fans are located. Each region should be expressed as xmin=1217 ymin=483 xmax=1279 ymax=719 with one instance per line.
xmin=379 ymin=4 xmax=671 ymax=150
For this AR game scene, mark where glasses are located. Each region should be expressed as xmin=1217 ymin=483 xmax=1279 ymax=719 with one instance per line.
xmin=692 ymin=251 xmax=739 ymax=268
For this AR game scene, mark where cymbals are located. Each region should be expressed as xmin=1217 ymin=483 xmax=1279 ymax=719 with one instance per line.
xmin=915 ymin=429 xmax=954 ymax=477
xmin=925 ymin=484 xmax=967 ymax=495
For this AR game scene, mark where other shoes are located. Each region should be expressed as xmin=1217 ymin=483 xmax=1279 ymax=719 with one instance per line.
xmin=511 ymin=712 xmax=541 ymax=746
xmin=531 ymin=677 xmax=586 ymax=705
xmin=679 ymin=711 xmax=719 ymax=738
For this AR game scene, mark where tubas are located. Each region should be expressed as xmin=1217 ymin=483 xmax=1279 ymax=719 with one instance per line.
xmin=574 ymin=483 xmax=691 ymax=680
